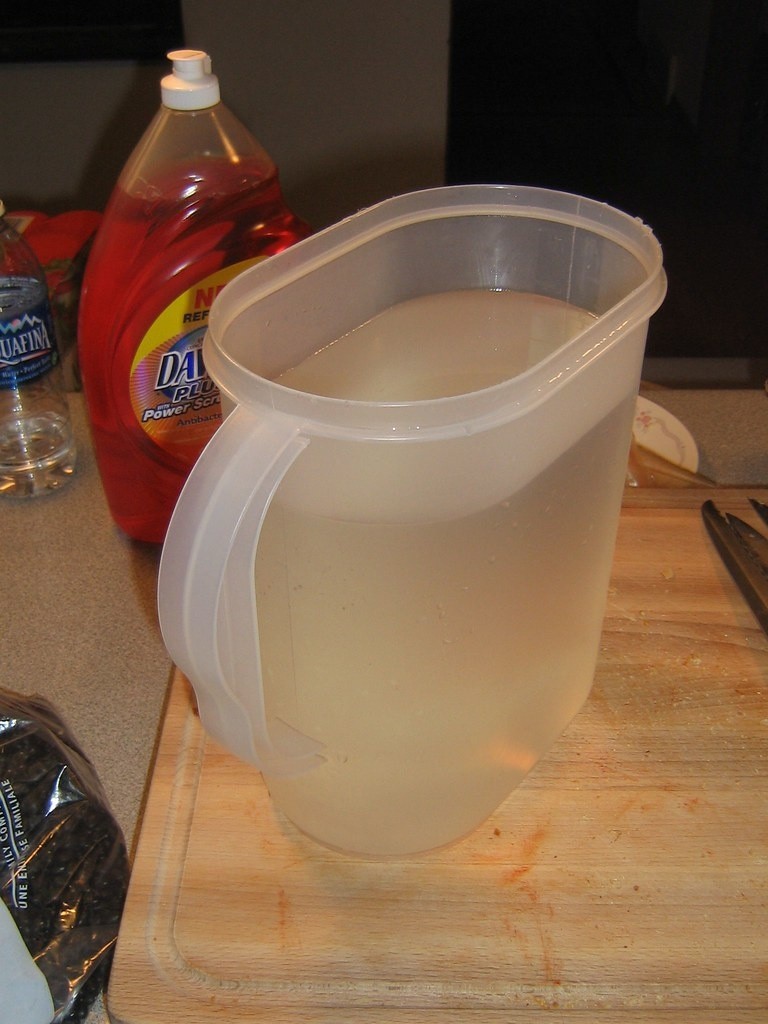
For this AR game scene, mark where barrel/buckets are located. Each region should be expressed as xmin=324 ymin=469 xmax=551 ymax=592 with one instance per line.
xmin=156 ymin=182 xmax=667 ymax=856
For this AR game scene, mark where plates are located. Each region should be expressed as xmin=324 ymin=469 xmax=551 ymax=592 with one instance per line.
xmin=623 ymin=392 xmax=700 ymax=493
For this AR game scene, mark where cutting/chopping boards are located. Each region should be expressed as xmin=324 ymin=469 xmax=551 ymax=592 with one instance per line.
xmin=101 ymin=474 xmax=768 ymax=1024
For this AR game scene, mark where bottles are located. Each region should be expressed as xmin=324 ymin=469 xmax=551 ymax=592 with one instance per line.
xmin=1 ymin=198 xmax=75 ymax=501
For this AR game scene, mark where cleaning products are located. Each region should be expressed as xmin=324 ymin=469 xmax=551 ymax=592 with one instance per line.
xmin=79 ymin=49 xmax=315 ymax=542
